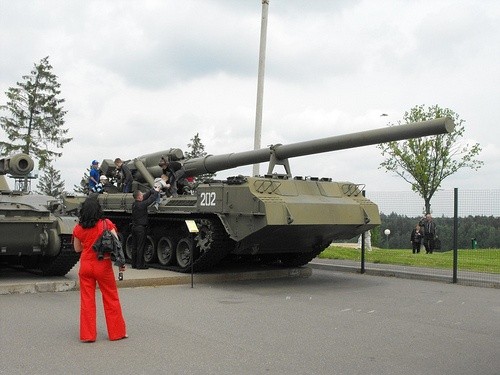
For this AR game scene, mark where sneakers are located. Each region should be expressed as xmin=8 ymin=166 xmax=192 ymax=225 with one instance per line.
xmin=137 ymin=266 xmax=149 ymax=269
xmin=132 ymin=264 xmax=138 ymax=268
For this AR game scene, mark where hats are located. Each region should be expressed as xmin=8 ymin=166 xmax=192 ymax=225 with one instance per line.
xmin=100 ymin=175 xmax=108 ymax=179
xmin=92 ymin=160 xmax=99 ymax=165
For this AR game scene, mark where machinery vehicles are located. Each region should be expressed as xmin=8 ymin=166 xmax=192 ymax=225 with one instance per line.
xmin=0 ymin=152 xmax=81 ymax=278
xmin=59 ymin=115 xmax=456 ymax=275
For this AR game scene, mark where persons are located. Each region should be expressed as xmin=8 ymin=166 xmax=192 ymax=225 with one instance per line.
xmin=89 ymin=160 xmax=104 ymax=195
xmin=114 ymin=158 xmax=133 ymax=193
xmin=73 ymin=195 xmax=129 ymax=342
xmin=151 ymin=174 xmax=170 ymax=210
xmin=419 ymin=214 xmax=438 ymax=254
xmin=358 ymin=230 xmax=372 ymax=254
xmin=131 ymin=186 xmax=160 ymax=270
xmin=410 ymin=224 xmax=424 ymax=254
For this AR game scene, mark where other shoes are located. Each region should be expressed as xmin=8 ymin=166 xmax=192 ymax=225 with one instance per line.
xmin=119 ymin=334 xmax=129 ymax=339
xmin=430 ymin=251 xmax=432 ymax=254
xmin=426 ymin=250 xmax=429 ymax=254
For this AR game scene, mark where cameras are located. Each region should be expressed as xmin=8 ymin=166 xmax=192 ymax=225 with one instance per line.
xmin=119 ymin=273 xmax=123 ymax=280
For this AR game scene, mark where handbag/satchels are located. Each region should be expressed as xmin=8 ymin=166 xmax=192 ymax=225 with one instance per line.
xmin=92 ymin=219 xmax=121 ymax=261
xmin=432 ymin=238 xmax=441 ymax=249
